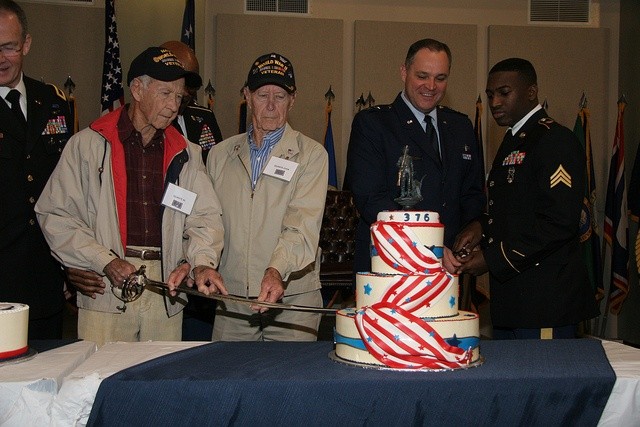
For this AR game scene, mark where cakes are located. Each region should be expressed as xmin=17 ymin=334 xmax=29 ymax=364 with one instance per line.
xmin=335 ymin=210 xmax=481 ymax=370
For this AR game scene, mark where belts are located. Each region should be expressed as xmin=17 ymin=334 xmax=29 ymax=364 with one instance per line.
xmin=126 ymin=247 xmax=161 ymax=260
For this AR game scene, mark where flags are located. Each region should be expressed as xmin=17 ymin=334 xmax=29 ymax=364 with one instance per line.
xmin=601 ymin=99 xmax=634 ymax=316
xmin=320 ymin=110 xmax=339 ymax=192
xmin=570 ymin=109 xmax=606 ymax=322
xmin=100 ymin=0 xmax=125 ymax=117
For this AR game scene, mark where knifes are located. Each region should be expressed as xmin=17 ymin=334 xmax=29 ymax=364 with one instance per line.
xmin=110 ymin=265 xmax=340 ymax=315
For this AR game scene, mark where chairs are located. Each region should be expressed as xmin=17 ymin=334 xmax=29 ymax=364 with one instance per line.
xmin=319 ymin=190 xmax=360 ymax=288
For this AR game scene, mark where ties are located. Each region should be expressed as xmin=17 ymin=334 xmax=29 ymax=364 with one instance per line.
xmin=5 ymin=89 xmax=26 ymax=127
xmin=495 ymin=128 xmax=512 ymax=167
xmin=424 ymin=114 xmax=441 ymax=165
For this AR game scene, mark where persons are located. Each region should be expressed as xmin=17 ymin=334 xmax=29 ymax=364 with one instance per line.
xmin=396 ymin=144 xmax=415 ymax=199
xmin=450 ymin=56 xmax=602 ymax=341
xmin=34 ymin=46 xmax=229 ymax=342
xmin=205 ymin=51 xmax=331 ymax=344
xmin=159 ymin=41 xmax=225 ymax=343
xmin=343 ymin=38 xmax=487 ymax=311
xmin=0 ymin=1 xmax=80 ymax=341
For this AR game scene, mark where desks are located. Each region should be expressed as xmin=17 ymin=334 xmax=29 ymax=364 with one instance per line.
xmin=50 ymin=340 xmax=640 ymax=427
xmin=0 ymin=339 xmax=99 ymax=427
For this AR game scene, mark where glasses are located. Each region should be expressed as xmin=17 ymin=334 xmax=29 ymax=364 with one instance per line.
xmin=0 ymin=41 xmax=23 ymax=58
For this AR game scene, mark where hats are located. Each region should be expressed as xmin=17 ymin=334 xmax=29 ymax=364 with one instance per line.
xmin=248 ymin=52 xmax=296 ymax=95
xmin=128 ymin=47 xmax=202 ymax=91
